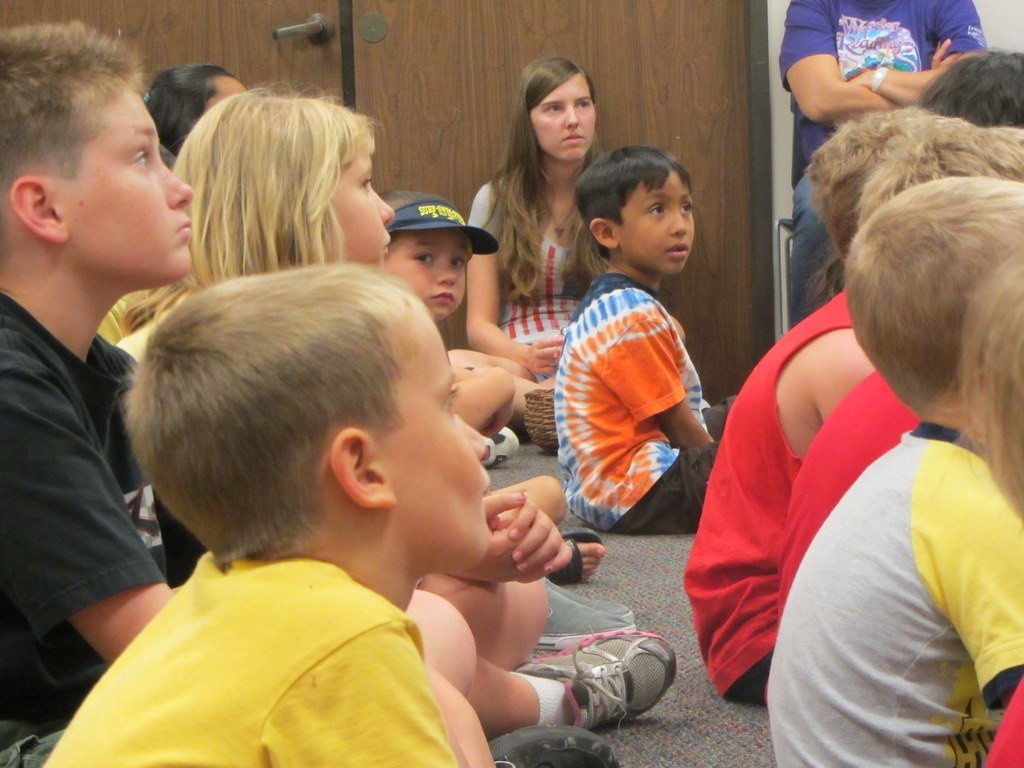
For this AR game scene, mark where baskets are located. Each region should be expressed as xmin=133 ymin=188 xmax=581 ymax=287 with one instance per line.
xmin=524 ymin=388 xmax=559 ymax=453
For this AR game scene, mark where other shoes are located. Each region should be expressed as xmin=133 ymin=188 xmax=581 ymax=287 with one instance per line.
xmin=483 ymin=426 xmax=520 ymax=467
xmin=533 ymin=582 xmax=637 ymax=651
xmin=487 ymin=723 xmax=620 ymax=768
xmin=543 ymin=578 xmax=635 ymax=623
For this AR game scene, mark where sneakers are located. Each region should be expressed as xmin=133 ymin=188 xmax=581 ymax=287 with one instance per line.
xmin=514 ymin=629 xmax=676 ymax=730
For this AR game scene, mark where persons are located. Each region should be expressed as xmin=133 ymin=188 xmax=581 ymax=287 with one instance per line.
xmin=43 ymin=263 xmax=624 ymax=768
xmin=173 ymin=82 xmax=677 ymax=732
xmin=141 ymin=62 xmax=247 ymax=173
xmin=777 ymin=0 xmax=989 ymax=332
xmin=0 ymin=21 xmax=196 ymax=768
xmin=684 ymin=50 xmax=1024 ymax=768
xmin=552 ymin=144 xmax=718 ymax=534
xmin=465 ymin=55 xmax=603 ymax=430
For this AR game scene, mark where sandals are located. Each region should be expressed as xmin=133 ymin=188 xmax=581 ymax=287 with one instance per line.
xmin=545 ymin=528 xmax=601 ymax=583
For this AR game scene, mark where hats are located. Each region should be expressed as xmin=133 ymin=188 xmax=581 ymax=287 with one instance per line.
xmin=387 ymin=199 xmax=499 ymax=255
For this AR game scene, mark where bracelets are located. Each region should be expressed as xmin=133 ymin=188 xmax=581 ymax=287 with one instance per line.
xmin=872 ymin=67 xmax=889 ymax=92
